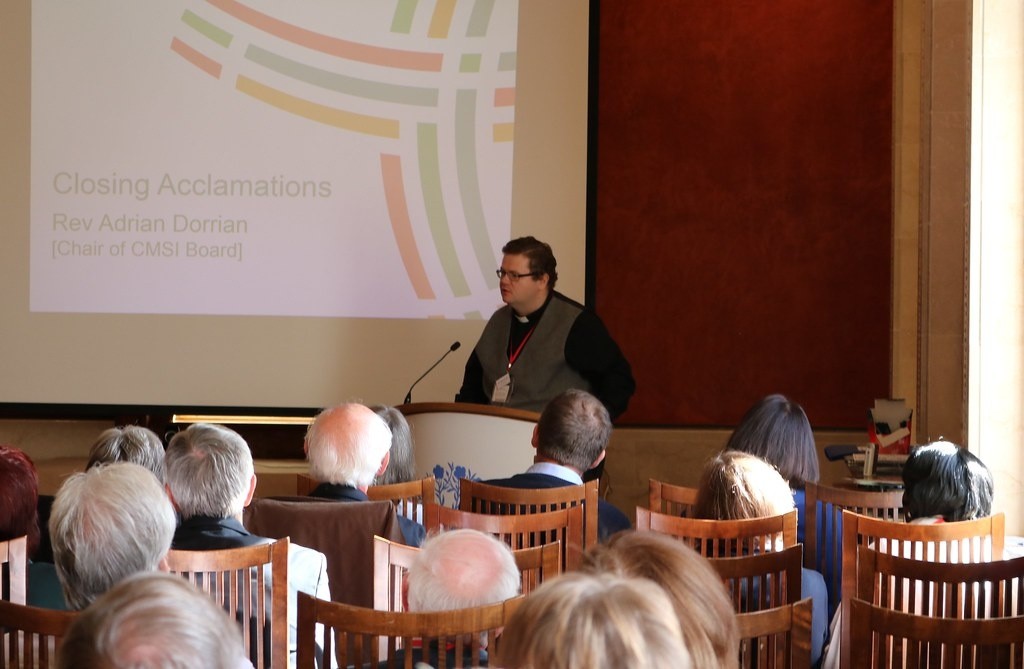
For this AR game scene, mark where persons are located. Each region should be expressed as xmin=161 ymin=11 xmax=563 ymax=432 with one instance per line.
xmin=463 ymin=390 xmax=631 ymax=539
xmin=87 ymin=425 xmax=166 ymax=486
xmin=493 ymin=572 xmax=694 ymax=669
xmin=372 ymin=529 xmax=521 ymax=669
xmin=62 ymin=570 xmax=256 ymax=669
xmin=578 ymin=531 xmax=740 ymax=669
xmin=304 ymin=403 xmax=425 ymax=546
xmin=167 ymin=423 xmax=339 ymax=669
xmin=870 ymin=440 xmax=1024 ymax=669
xmin=730 ymin=395 xmax=843 ymax=617
xmin=455 ymin=236 xmax=635 ymax=492
xmin=696 ymin=452 xmax=828 ymax=669
xmin=49 ymin=461 xmax=176 ymax=606
xmin=0 ymin=444 xmax=40 ymax=566
xmin=374 ymin=408 xmax=443 ymax=534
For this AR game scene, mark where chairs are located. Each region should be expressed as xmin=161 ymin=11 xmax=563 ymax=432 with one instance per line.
xmin=0 ymin=474 xmax=1024 ymax=669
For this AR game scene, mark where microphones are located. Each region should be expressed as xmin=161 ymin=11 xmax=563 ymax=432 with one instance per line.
xmin=403 ymin=341 xmax=461 ymax=404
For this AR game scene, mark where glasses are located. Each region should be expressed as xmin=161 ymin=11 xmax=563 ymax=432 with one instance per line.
xmin=495 ymin=267 xmax=547 ymax=282
